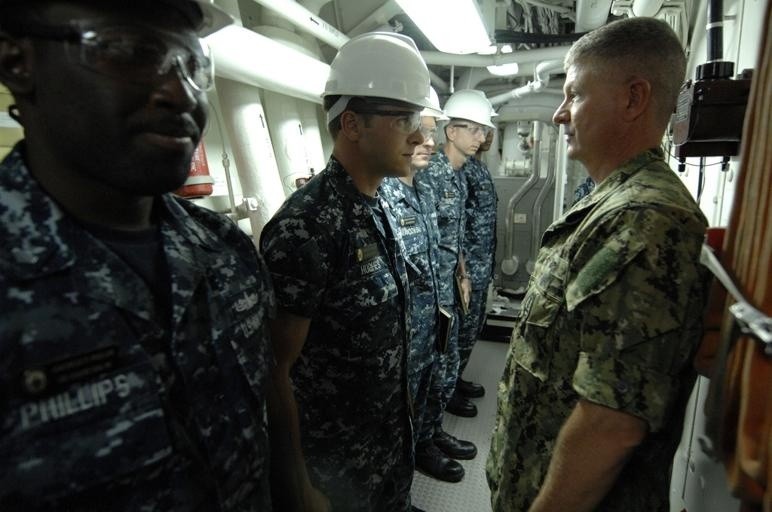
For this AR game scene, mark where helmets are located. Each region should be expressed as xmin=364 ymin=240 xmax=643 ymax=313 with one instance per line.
xmin=419 ymin=84 xmax=499 ymax=129
xmin=319 ymin=31 xmax=444 ymax=115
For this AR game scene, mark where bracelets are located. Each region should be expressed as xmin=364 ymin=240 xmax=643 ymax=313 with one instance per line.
xmin=460 ymin=274 xmax=470 ymax=280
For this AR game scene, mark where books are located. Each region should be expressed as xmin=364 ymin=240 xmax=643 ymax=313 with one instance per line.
xmin=452 ymin=273 xmax=471 ymax=316
xmin=435 ymin=304 xmax=452 ymax=354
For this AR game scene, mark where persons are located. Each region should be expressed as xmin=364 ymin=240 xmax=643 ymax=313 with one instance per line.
xmin=381 ymin=81 xmax=451 ymax=453
xmin=1 ymin=0 xmax=281 ymax=511
xmin=482 ymin=12 xmax=710 ymax=512
xmin=414 ymin=86 xmax=497 ymax=484
xmin=444 ymin=89 xmax=500 ymax=419
xmin=258 ymin=30 xmax=442 ymax=512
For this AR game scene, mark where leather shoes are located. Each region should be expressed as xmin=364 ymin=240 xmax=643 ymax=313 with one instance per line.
xmin=458 ymin=379 xmax=485 ymax=398
xmin=445 ymin=397 xmax=478 ymax=418
xmin=413 ymin=442 xmax=465 ymax=484
xmin=434 ymin=432 xmax=477 ymax=460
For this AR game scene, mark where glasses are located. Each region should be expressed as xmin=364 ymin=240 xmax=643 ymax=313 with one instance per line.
xmin=338 ymin=109 xmax=421 ymax=135
xmin=420 ymin=127 xmax=441 ymax=141
xmin=10 ymin=18 xmax=215 ymax=95
xmin=452 ymin=124 xmax=488 ymax=137
